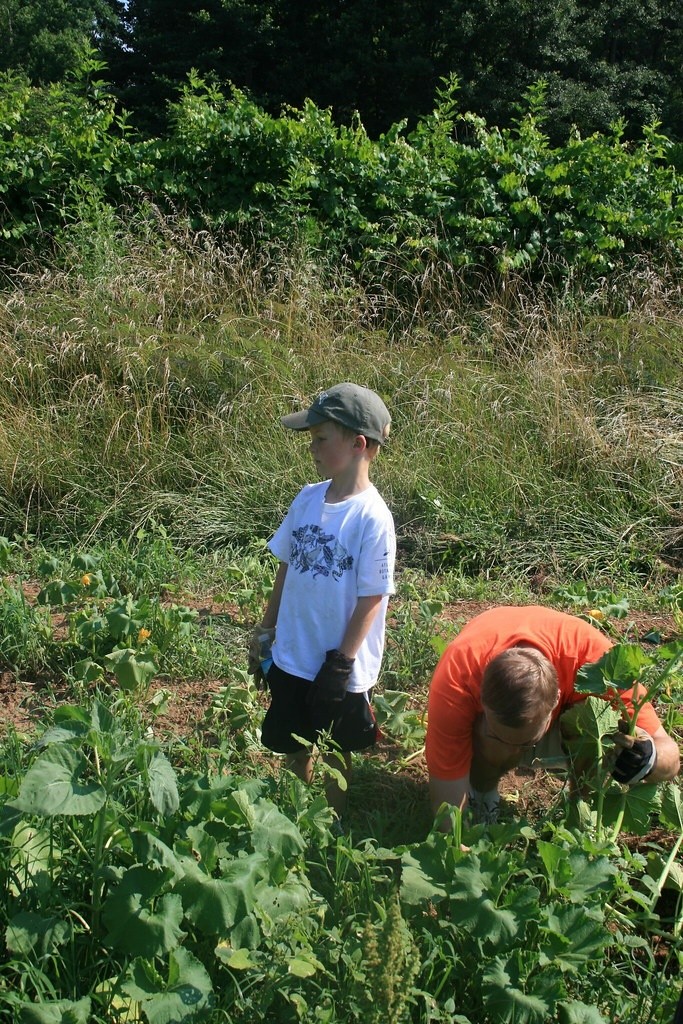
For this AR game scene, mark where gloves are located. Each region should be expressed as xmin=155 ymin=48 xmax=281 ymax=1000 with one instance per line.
xmin=248 ymin=623 xmax=275 ymax=691
xmin=304 ymin=650 xmax=354 ymax=713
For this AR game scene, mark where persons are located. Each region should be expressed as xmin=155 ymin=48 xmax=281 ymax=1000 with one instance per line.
xmin=248 ymin=383 xmax=397 ymax=818
xmin=424 ymin=606 xmax=681 ymax=836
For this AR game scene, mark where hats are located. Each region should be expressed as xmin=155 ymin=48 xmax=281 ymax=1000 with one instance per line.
xmin=280 ymin=383 xmax=392 ymax=445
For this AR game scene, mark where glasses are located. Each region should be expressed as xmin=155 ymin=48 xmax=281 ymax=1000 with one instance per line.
xmin=482 ymin=714 xmax=548 ymax=748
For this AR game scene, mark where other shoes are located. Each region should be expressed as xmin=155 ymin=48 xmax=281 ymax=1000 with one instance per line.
xmin=469 ymin=785 xmax=500 ymax=827
